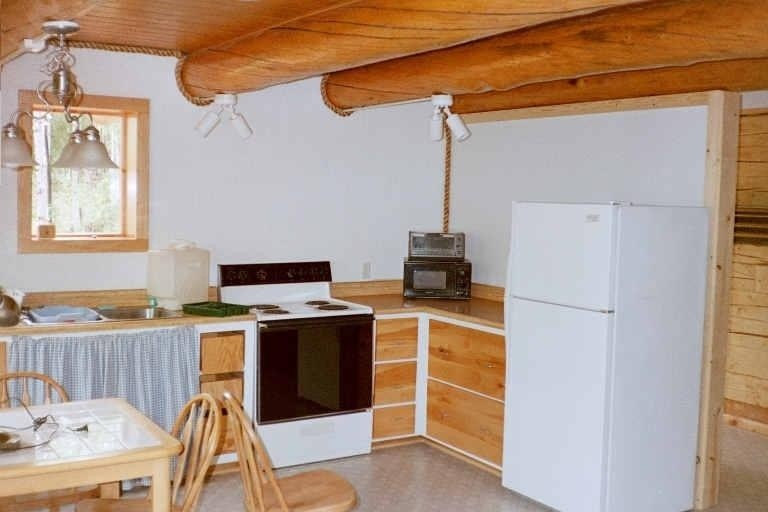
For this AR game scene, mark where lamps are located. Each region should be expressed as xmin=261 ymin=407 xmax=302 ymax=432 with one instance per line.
xmin=194 ymin=93 xmax=254 ymax=139
xmin=0 ymin=20 xmax=119 ymax=169
xmin=429 ymin=94 xmax=471 ymax=143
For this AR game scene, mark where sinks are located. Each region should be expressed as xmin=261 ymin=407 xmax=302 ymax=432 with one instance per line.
xmin=94 ymin=305 xmax=173 ymax=320
xmin=24 ymin=311 xmax=104 ymax=323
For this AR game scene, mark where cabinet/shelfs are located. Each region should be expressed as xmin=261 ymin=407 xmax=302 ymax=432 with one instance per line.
xmin=2 ymin=297 xmax=256 ymax=492
xmin=373 ymin=295 xmax=506 ymax=481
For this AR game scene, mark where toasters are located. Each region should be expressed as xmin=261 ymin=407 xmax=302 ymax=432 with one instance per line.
xmin=407 ymin=230 xmax=465 ymax=262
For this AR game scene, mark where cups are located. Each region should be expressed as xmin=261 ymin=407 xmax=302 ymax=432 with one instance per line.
xmin=37 ymin=202 xmax=56 ymax=238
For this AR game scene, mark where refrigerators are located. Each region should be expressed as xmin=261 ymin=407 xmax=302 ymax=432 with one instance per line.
xmin=495 ymin=198 xmax=709 ymax=512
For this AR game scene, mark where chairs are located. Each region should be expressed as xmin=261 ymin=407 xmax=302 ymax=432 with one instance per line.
xmin=222 ymin=390 xmax=359 ymax=512
xmin=0 ymin=371 xmax=99 ymax=512
xmin=71 ymin=392 xmax=222 ymax=512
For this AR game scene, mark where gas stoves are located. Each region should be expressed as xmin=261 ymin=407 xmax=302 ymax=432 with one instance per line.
xmin=239 ymin=297 xmax=373 ymax=320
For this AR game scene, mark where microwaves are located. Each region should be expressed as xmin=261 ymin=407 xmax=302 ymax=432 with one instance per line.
xmin=402 ymin=260 xmax=473 ymax=302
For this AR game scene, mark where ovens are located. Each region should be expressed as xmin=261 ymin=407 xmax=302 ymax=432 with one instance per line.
xmin=257 ymin=317 xmax=375 ymax=424
